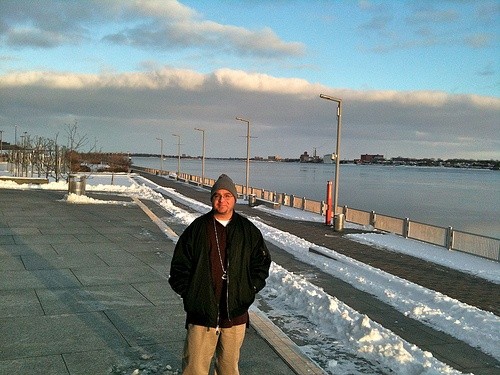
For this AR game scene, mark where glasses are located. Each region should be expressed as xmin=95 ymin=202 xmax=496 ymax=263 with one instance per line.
xmin=213 ymin=193 xmax=234 ymax=200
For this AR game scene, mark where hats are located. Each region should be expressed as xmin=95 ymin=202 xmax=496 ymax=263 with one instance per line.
xmin=210 ymin=174 xmax=237 ymax=202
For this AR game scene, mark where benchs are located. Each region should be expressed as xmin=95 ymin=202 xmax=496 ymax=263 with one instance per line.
xmin=169 ymin=175 xmax=177 ymax=179
xmin=203 ymin=184 xmax=213 ymax=191
xmin=189 ymin=180 xmax=201 ymax=186
xmin=178 ymin=178 xmax=187 ymax=182
xmin=237 ymin=192 xmax=244 ymax=199
xmin=256 ymin=199 xmax=281 ymax=210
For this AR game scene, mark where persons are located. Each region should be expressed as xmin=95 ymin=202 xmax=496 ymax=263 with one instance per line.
xmin=168 ymin=174 xmax=271 ymax=375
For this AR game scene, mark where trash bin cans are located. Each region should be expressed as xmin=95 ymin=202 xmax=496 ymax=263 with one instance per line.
xmin=68 ymin=174 xmax=86 ymax=198
xmin=249 ymin=194 xmax=256 ymax=206
xmin=334 ymin=214 xmax=345 ymax=231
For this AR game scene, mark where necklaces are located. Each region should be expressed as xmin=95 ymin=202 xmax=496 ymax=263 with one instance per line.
xmin=212 ymin=211 xmax=227 ymax=280
